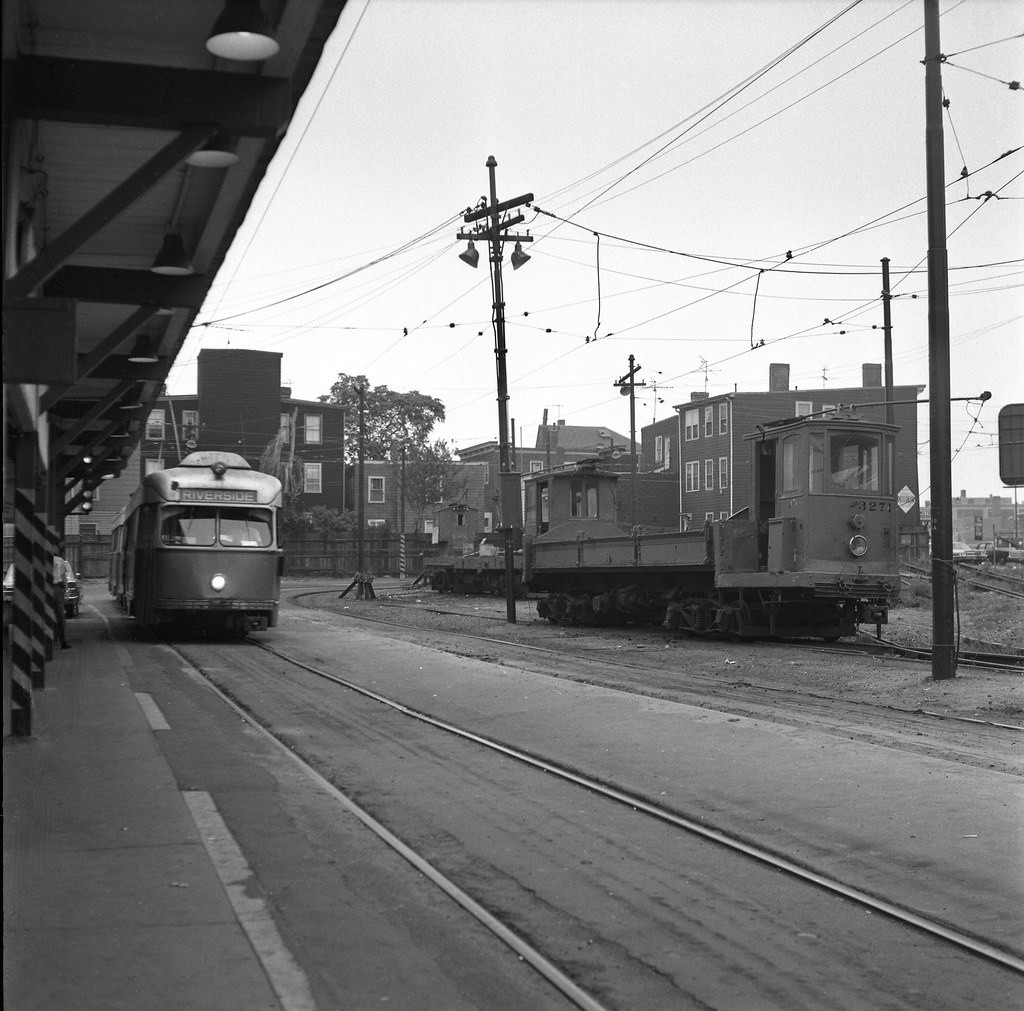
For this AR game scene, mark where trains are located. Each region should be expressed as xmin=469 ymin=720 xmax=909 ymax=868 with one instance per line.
xmin=108 ymin=396 xmax=283 ymax=641
xmin=523 ymin=390 xmax=992 ymax=640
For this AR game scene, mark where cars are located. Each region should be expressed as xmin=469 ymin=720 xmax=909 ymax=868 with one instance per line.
xmin=3 ymin=556 xmax=83 ymax=618
xmin=953 ymin=540 xmax=1024 ymax=565
